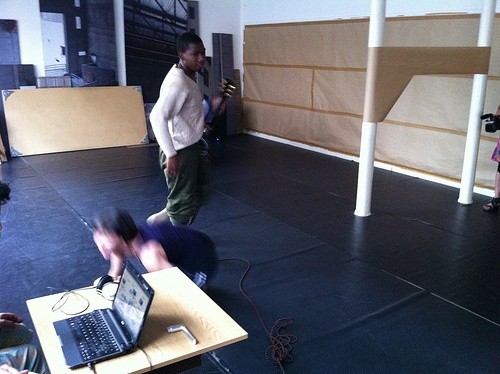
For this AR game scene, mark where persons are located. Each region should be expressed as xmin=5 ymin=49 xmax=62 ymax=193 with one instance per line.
xmin=482 ymin=104 xmax=500 ymax=212
xmin=91 ymin=208 xmax=219 ymax=289
xmin=146 ymin=31 xmax=228 ymax=228
xmin=0 ymin=313 xmax=48 ymax=374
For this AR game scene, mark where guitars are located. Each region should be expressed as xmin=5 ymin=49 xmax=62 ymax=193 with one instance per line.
xmin=203 ymin=78 xmax=236 ymax=140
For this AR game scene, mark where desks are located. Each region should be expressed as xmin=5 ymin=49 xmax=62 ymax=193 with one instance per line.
xmin=25 ymin=266 xmax=248 ymax=374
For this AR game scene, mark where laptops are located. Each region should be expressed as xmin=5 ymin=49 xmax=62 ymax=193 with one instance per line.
xmin=53 ymin=259 xmax=154 ymax=369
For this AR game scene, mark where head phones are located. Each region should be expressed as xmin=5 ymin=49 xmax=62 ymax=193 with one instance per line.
xmin=93 ymin=274 xmax=121 ymax=300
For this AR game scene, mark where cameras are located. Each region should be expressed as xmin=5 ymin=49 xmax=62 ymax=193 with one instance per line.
xmin=481 ymin=113 xmax=500 ymax=132
